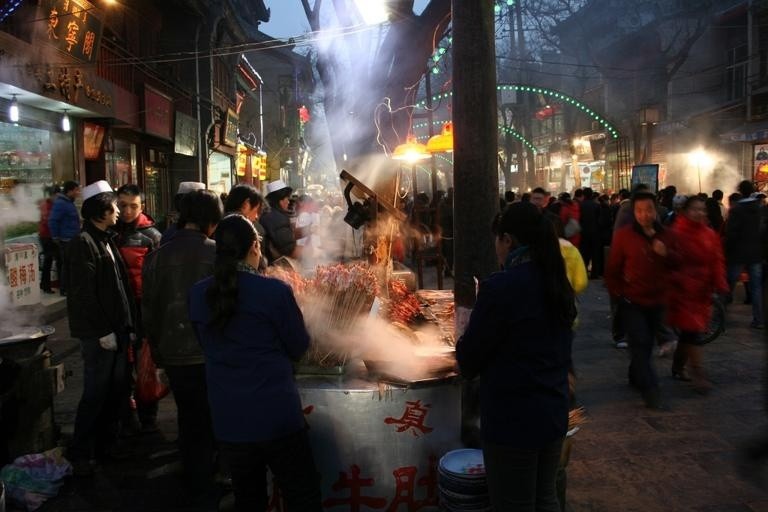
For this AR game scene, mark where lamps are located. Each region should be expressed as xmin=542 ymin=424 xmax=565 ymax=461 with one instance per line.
xmin=9 ymin=93 xmax=19 ymax=121
xmin=62 ymin=109 xmax=70 ymax=131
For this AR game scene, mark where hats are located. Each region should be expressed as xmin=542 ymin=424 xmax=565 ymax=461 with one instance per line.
xmin=267 ymin=178 xmax=290 ymax=193
xmin=80 ymin=179 xmax=114 ymax=204
xmin=176 ymin=181 xmax=206 ymax=194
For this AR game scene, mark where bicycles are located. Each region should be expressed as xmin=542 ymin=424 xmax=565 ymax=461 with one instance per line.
xmin=671 ymin=293 xmax=727 ymax=347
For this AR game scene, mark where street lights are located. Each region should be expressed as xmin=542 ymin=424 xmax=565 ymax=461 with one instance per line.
xmin=638 ymin=98 xmax=660 ymax=165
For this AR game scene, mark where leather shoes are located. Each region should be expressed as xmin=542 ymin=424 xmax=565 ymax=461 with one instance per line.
xmin=647 ymin=398 xmax=670 ymax=411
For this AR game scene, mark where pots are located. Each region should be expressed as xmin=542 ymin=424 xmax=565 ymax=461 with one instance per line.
xmin=1 ymin=324 xmax=55 ymax=364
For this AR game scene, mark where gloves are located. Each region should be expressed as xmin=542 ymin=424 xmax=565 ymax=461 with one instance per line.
xmin=99 ymin=332 xmax=118 ymax=352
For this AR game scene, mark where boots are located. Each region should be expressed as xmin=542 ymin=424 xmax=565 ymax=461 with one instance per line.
xmin=686 ymin=345 xmax=711 ymax=390
xmin=671 ymin=343 xmax=691 ymax=381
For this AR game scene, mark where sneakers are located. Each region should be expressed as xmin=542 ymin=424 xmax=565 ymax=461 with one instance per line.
xmin=751 ymin=321 xmax=763 ymax=328
xmin=119 ymin=422 xmax=140 ymax=437
xmin=615 ymin=338 xmax=627 ymax=348
xmin=142 ymin=420 xmax=156 ymax=434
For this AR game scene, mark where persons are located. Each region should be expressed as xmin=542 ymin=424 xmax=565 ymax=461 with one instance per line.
xmin=67 ymin=180 xmax=168 ymax=457
xmin=503 ymin=186 xmax=678 ymax=347
xmin=452 ymin=203 xmax=576 ymax=510
xmin=699 ymin=181 xmax=767 ymax=328
xmin=401 ymin=186 xmax=454 ymax=276
xmin=38 ymin=180 xmax=79 ymax=297
xmin=665 ymin=196 xmax=728 ymax=388
xmin=609 ymin=193 xmax=682 ymax=410
xmin=179 ymin=181 xmax=312 ymax=269
xmin=140 ymin=192 xmax=220 ymax=506
xmin=185 ymin=213 xmax=321 ymax=509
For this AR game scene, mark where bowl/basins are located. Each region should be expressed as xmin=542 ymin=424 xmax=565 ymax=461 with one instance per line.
xmin=440 ymin=447 xmax=486 ymax=478
